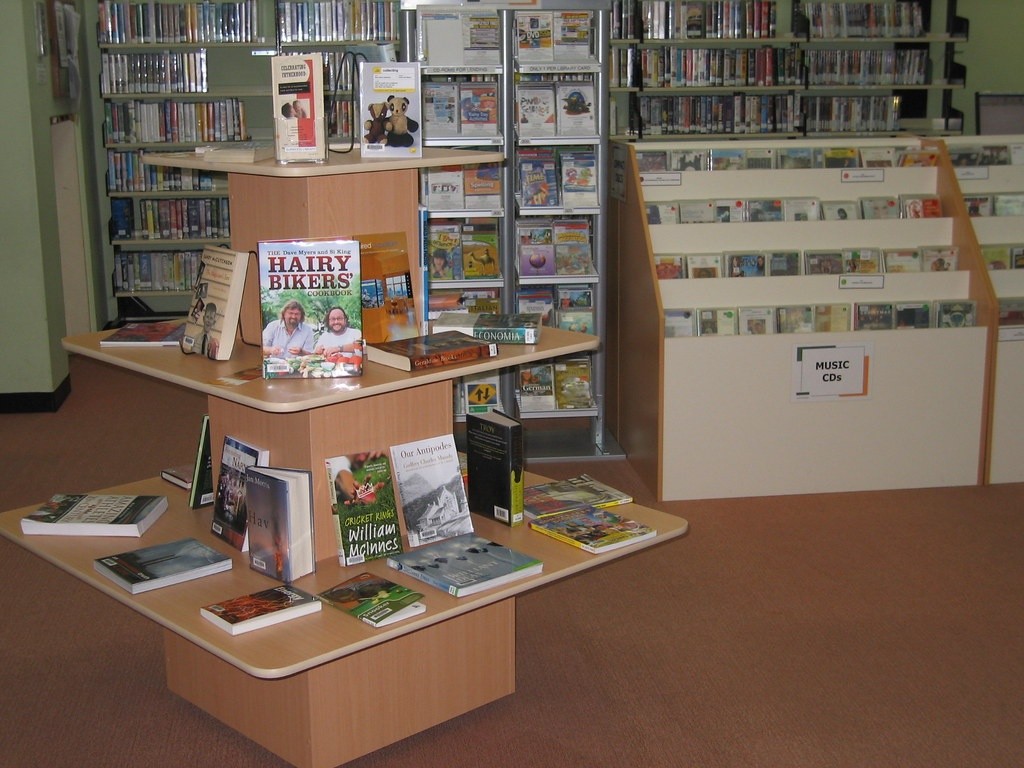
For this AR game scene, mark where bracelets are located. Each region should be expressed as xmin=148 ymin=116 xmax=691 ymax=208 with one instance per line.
xmin=299 ymin=348 xmax=302 ymax=355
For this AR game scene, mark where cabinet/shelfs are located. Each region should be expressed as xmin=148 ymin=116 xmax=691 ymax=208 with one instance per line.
xmin=0 ymin=0 xmax=1024 ymax=768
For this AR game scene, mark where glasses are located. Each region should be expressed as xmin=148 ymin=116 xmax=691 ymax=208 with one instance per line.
xmin=330 ymin=317 xmax=345 ymax=321
xmin=288 ymin=312 xmax=300 ymax=316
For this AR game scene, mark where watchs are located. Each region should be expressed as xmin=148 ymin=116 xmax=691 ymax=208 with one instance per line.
xmin=339 ymin=345 xmax=343 ymax=352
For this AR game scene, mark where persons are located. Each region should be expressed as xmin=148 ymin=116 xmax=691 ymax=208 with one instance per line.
xmin=282 ymin=103 xmax=296 ymax=118
xmin=192 ymin=301 xmax=202 ymax=323
xmin=337 ymin=339 xmax=362 ymax=369
xmin=192 ymin=302 xmax=216 ymax=355
xmin=312 ymin=307 xmax=361 ymax=357
xmin=263 ymin=301 xmax=313 ymax=358
xmin=293 ymin=101 xmax=306 ymax=118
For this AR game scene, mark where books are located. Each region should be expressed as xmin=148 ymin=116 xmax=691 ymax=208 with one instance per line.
xmin=366 ymin=329 xmax=498 ymax=372
xmin=17 ymin=413 xmax=213 ymax=535
xmin=315 ymin=572 xmax=427 ymax=629
xmin=432 ymin=312 xmax=544 ymax=345
xmin=530 ymin=505 xmax=658 ymax=552
xmin=325 ymin=449 xmax=405 ymax=567
xmin=607 ymin=0 xmax=1024 ymax=337
xmin=91 ymin=536 xmax=233 ymax=594
xmin=520 ymin=472 xmax=634 ymax=518
xmin=199 ymin=585 xmax=323 ymax=636
xmin=390 ymin=532 xmax=544 ymax=598
xmin=240 ymin=464 xmax=317 ymax=583
xmin=466 ymin=409 xmax=524 ymax=528
xmin=179 ymin=243 xmax=249 ymax=361
xmin=35 ymin=1 xmax=602 ymax=426
xmin=390 ymin=432 xmax=475 ymax=547
